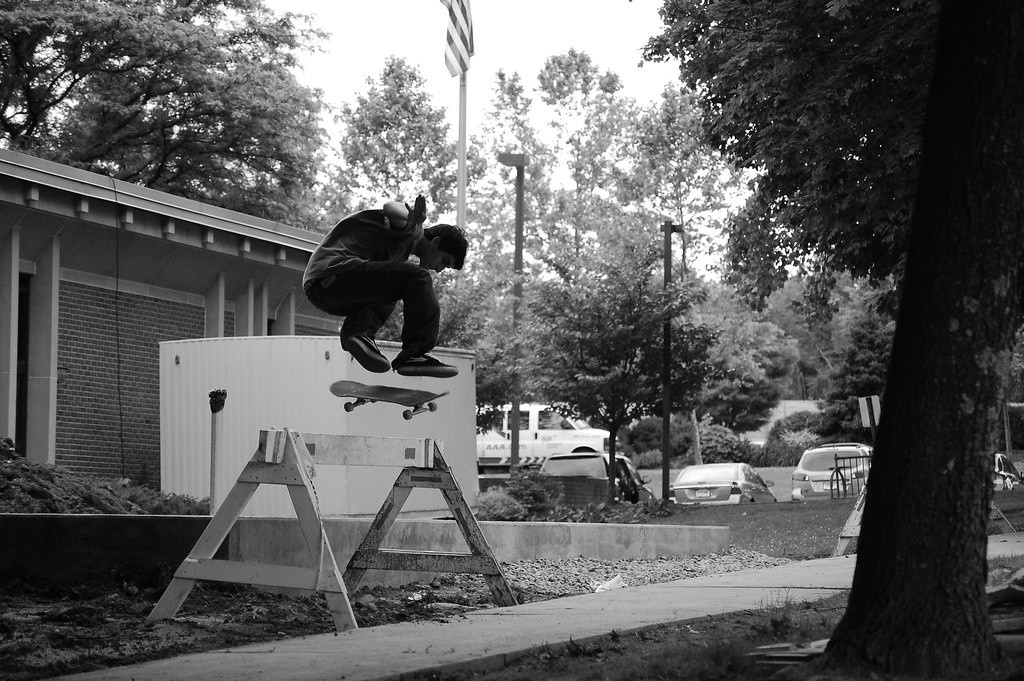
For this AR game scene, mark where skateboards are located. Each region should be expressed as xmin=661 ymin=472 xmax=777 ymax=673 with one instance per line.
xmin=331 ymin=380 xmax=450 ymax=421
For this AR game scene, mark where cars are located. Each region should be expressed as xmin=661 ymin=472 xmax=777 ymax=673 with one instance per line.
xmin=995 ymin=453 xmax=1024 ymax=487
xmin=789 ymin=441 xmax=874 ymax=502
xmin=668 ymin=461 xmax=779 ymax=505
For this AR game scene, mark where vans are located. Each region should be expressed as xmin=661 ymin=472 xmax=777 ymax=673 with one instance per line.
xmin=476 ymin=401 xmax=625 ymax=464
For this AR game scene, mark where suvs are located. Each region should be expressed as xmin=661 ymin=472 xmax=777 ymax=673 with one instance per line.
xmin=536 ymin=452 xmax=655 ymax=505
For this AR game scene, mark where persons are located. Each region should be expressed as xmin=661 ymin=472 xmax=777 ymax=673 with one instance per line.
xmin=301 ymin=194 xmax=469 ymax=378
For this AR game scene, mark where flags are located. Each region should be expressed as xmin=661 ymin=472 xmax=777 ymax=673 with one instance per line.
xmin=438 ymin=0 xmax=475 ymax=78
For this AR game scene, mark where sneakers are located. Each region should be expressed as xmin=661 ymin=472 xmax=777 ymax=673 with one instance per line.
xmin=345 ymin=332 xmax=391 ymax=373
xmin=397 ymin=352 xmax=459 ymax=378
